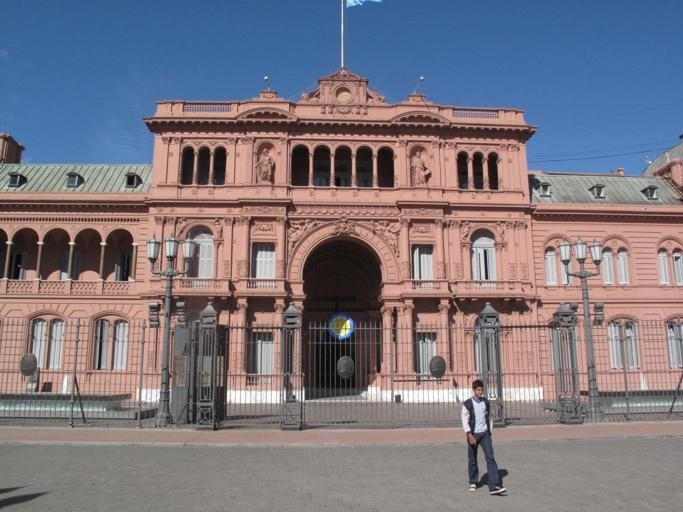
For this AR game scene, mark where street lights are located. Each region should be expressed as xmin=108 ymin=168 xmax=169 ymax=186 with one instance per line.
xmin=559 ymin=235 xmax=605 ymax=423
xmin=146 ymin=232 xmax=197 ymax=428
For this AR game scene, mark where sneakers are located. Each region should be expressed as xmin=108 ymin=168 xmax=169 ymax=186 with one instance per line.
xmin=469 ymin=483 xmax=477 ymax=491
xmin=488 ymin=486 xmax=507 ymax=495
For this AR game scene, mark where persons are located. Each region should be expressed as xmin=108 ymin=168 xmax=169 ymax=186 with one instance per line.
xmin=253 ymin=147 xmax=275 ymax=184
xmin=409 ymin=150 xmax=430 ymax=186
xmin=460 ymin=378 xmax=506 ymax=495
